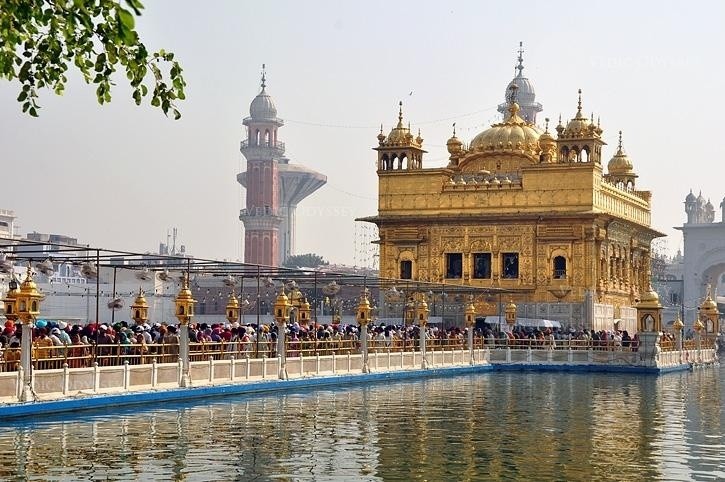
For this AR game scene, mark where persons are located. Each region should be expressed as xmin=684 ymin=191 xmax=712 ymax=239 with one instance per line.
xmin=1 ymin=317 xmax=696 ymax=372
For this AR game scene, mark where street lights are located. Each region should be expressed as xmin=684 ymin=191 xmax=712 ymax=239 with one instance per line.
xmin=672 ymin=313 xmax=703 ymax=363
xmin=12 ymin=264 xmax=50 ymax=404
xmin=226 ymin=285 xmax=241 ymax=326
xmin=1 ymin=282 xmax=23 ymax=324
xmin=127 ymin=282 xmax=152 ymax=327
xmin=172 ymin=277 xmax=201 ymax=389
xmin=271 ymin=282 xmax=517 ymax=385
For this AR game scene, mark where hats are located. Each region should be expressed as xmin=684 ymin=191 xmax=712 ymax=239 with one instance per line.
xmin=4 ymin=319 xmax=270 ymax=335
